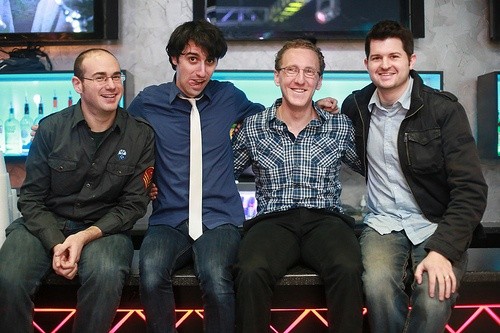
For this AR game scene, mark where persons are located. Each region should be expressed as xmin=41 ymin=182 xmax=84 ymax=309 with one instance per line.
xmin=30 ymin=20 xmax=338 ymax=331
xmin=1 ymin=49 xmax=158 ymax=333
xmin=341 ymin=20 xmax=488 ymax=333
xmin=149 ymin=39 xmax=366 ymax=332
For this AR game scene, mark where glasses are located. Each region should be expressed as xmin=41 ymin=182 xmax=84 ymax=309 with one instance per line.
xmin=79 ymin=75 xmax=126 ymax=84
xmin=278 ymin=67 xmax=321 ymax=78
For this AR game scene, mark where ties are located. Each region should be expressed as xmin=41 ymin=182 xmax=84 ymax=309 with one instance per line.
xmin=175 ymin=93 xmax=205 ymax=240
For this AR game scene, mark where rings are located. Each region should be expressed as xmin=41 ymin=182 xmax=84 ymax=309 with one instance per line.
xmin=444 ymin=276 xmax=450 ymax=283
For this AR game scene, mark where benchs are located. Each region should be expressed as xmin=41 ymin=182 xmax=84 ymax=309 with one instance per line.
xmin=32 ymin=246 xmax=500 ymax=333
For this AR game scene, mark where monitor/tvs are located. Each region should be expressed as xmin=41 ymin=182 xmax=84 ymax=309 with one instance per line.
xmin=0 ymin=0 xmax=119 ymax=46
xmin=191 ymin=0 xmax=427 ymax=42
xmin=0 ymin=70 xmax=127 ymax=163
xmin=208 ymin=69 xmax=443 ymax=184
